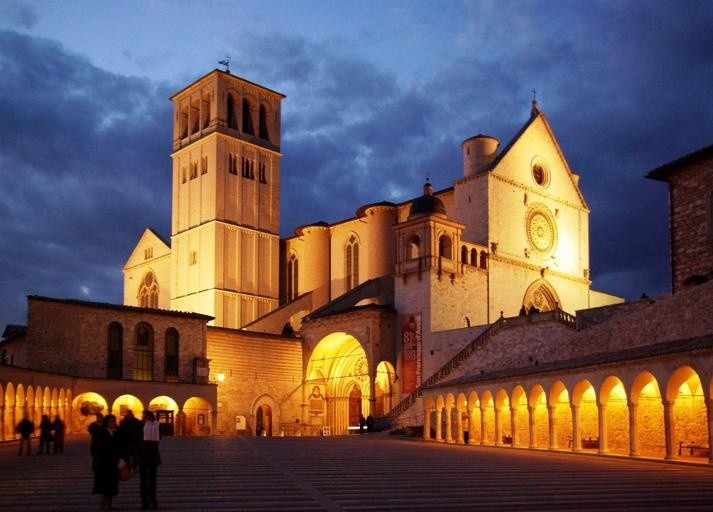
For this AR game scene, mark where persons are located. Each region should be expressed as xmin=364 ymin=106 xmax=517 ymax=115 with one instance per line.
xmin=641 ymin=294 xmax=649 ymax=298
xmin=366 ymin=414 xmax=373 ymax=432
xmin=359 ymin=414 xmax=365 ymax=433
xmin=529 ymin=306 xmax=539 ymax=314
xmin=15 ymin=412 xmax=33 ymax=456
xmin=37 ymin=415 xmax=51 ymax=454
xmin=51 ymin=415 xmax=64 ymax=454
xmin=519 ymin=305 xmax=526 ymax=316
xmin=88 ymin=409 xmax=160 ymax=512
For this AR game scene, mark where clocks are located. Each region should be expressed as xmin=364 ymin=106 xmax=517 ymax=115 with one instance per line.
xmin=527 ymin=207 xmax=555 ymax=256
